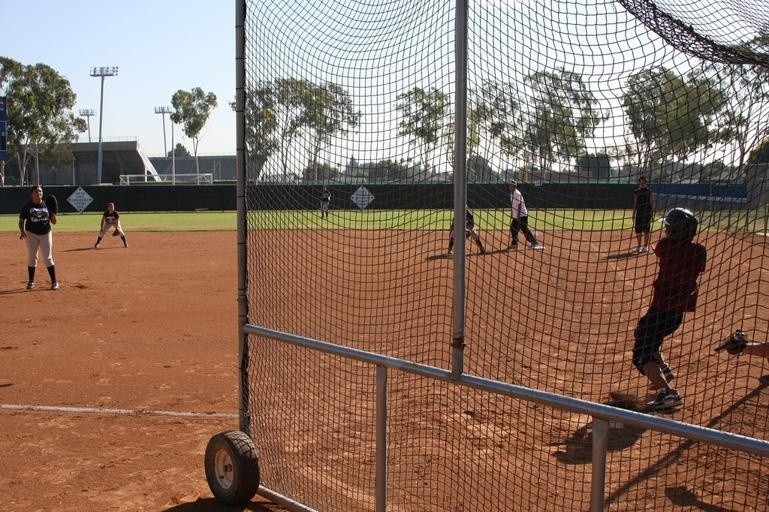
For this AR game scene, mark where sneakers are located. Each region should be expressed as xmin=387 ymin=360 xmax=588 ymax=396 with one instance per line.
xmin=95 ymin=244 xmax=99 ymax=248
xmin=648 ymin=363 xmax=676 ymax=390
xmin=27 ymin=282 xmax=35 ymax=289
xmin=631 ymin=246 xmax=648 ymax=254
xmin=51 ymin=282 xmax=58 ymax=290
xmin=448 ymin=250 xmax=452 ymax=255
xmin=508 ymin=241 xmax=539 ymax=249
xmin=480 ymin=247 xmax=486 ymax=254
xmin=124 ymin=241 xmax=129 ymax=247
xmin=644 ymin=388 xmax=684 ymax=413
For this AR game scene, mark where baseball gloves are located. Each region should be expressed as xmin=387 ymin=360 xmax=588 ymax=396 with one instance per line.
xmin=112 ymin=229 xmax=120 ymax=235
xmin=45 ymin=196 xmax=58 ymax=214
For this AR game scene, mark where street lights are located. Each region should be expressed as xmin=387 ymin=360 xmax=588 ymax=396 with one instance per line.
xmin=90 ymin=65 xmax=120 ymax=186
xmin=79 ymin=109 xmax=97 ymax=142
xmin=154 ymin=106 xmax=171 ymax=160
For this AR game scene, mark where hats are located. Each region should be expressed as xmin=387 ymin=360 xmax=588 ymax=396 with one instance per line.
xmin=658 ymin=207 xmax=699 ymax=227
xmin=510 ymin=180 xmax=517 ymax=186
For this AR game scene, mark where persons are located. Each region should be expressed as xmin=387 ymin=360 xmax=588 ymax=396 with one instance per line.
xmin=17 ymin=184 xmax=60 ymax=291
xmin=630 ymin=204 xmax=709 ymax=413
xmin=714 ymin=325 xmax=769 ymax=374
xmin=630 ymin=176 xmax=655 ymax=255
xmin=93 ymin=201 xmax=129 ymax=250
xmin=319 ymin=184 xmax=332 ymax=218
xmin=503 ymin=177 xmax=540 ymax=251
xmin=445 ymin=201 xmax=486 ymax=259
